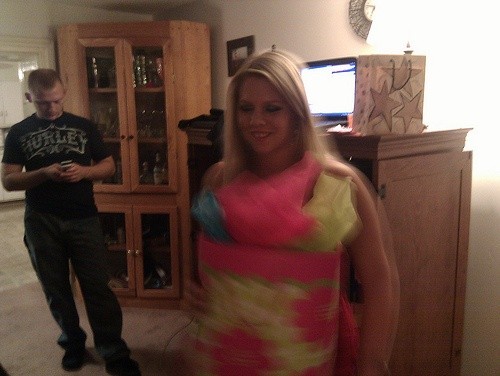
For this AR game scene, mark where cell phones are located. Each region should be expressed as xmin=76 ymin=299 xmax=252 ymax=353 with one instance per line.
xmin=59 ymin=160 xmax=73 ymax=174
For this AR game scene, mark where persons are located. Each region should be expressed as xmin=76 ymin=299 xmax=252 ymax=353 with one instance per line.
xmin=183 ymin=49 xmax=390 ymax=376
xmin=1 ymin=68 xmax=142 ymax=376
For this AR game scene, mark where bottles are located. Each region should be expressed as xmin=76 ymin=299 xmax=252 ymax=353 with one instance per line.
xmin=133 ymin=56 xmax=163 ymax=88
xmin=153 ymin=151 xmax=168 ymax=184
xmin=88 ymin=56 xmax=100 ymax=88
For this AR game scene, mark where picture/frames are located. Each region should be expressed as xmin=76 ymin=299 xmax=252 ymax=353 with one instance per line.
xmin=226 ymin=36 xmax=255 ymax=77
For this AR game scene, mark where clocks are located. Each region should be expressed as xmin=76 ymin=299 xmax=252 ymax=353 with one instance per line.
xmin=348 ymin=0 xmax=376 ymax=39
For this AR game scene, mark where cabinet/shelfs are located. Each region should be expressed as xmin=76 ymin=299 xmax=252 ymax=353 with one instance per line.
xmin=178 ymin=120 xmax=473 ymax=376
xmin=55 ymin=19 xmax=213 ymax=309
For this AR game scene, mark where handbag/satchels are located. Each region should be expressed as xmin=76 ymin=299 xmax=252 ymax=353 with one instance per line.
xmin=352 ymin=55 xmax=426 ymax=136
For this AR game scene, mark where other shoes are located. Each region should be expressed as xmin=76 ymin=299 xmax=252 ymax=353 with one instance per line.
xmin=106 ymin=360 xmax=142 ymax=376
xmin=61 ymin=343 xmax=85 ymax=372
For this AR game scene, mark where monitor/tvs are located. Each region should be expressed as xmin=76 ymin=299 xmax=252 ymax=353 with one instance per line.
xmin=298 ymin=56 xmax=356 ymax=128
